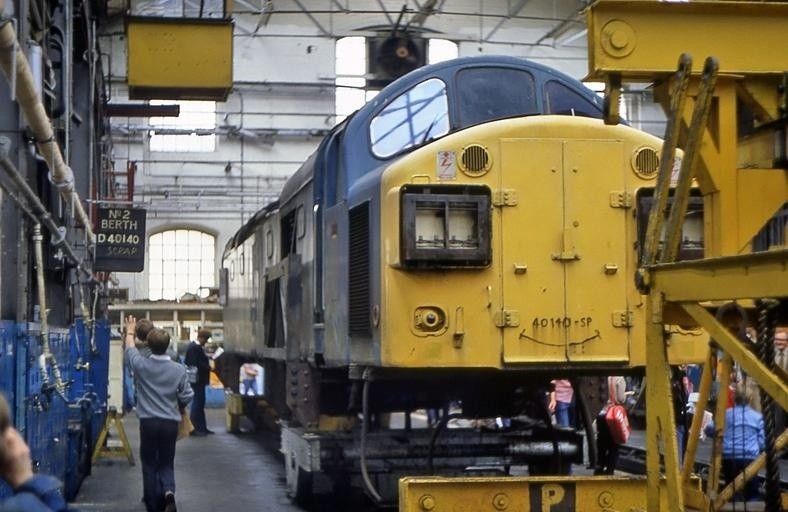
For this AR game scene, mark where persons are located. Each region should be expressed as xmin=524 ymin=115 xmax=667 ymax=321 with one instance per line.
xmin=179 ymin=328 xmax=215 ymax=437
xmin=594 ymin=372 xmax=637 ymax=474
xmin=123 ymin=314 xmax=196 ymax=512
xmin=664 ymin=325 xmax=787 ymax=500
xmin=549 ymin=378 xmax=580 ymax=427
xmin=242 ymin=363 xmax=262 ymax=397
xmin=0 ymin=392 xmax=67 ymax=511
xmin=131 ymin=317 xmax=184 ymax=366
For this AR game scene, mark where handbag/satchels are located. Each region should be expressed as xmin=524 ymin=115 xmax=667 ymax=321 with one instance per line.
xmin=186 ymin=366 xmax=198 ymax=382
xmin=606 ymin=404 xmax=631 ymax=445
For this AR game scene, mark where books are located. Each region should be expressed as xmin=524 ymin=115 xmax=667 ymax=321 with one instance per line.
xmin=212 ymin=346 xmax=225 ymax=360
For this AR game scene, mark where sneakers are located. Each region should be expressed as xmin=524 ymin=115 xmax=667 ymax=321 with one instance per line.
xmin=188 ymin=427 xmax=216 ymax=437
xmin=163 ymin=490 xmax=177 ymax=512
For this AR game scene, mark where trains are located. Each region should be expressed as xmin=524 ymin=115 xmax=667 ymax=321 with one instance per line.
xmin=220 ymin=54 xmax=712 ymax=510
